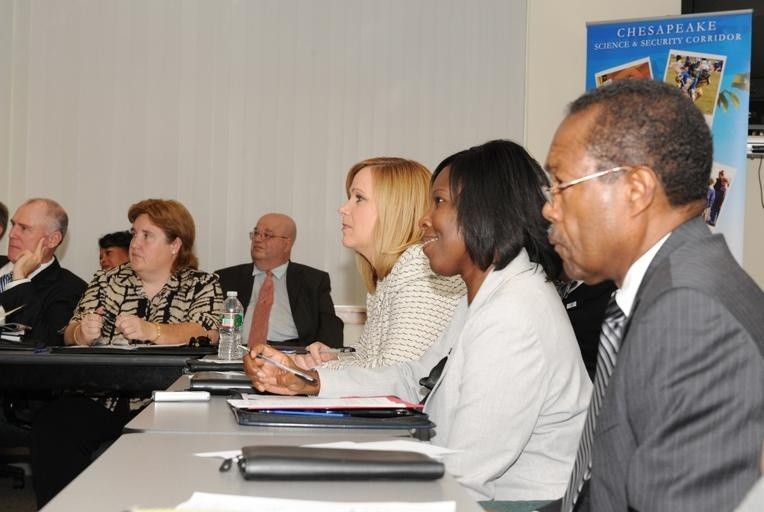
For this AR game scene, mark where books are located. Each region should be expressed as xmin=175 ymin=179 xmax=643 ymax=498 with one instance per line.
xmin=220 ymin=443 xmax=444 ymax=481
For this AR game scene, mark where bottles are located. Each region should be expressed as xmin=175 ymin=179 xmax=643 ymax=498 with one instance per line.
xmin=218 ymin=291 xmax=244 ymax=361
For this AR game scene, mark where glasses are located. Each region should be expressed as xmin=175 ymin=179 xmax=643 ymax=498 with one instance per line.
xmin=250 ymin=231 xmax=289 ymax=241
xmin=540 ymin=163 xmax=633 ymax=209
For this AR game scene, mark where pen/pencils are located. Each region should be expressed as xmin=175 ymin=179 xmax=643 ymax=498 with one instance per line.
xmin=238 ymin=344 xmax=318 ymax=384
xmin=339 ymin=409 xmax=415 ymax=417
xmin=260 ymin=408 xmax=351 ymax=418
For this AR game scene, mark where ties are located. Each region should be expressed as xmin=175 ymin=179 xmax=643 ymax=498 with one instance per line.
xmin=562 ymin=290 xmax=630 ymax=510
xmin=0 ymin=272 xmax=14 ymax=294
xmin=246 ymin=272 xmax=274 ymax=349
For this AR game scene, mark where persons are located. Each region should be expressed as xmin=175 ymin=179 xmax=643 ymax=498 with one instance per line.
xmin=702 ymin=171 xmax=729 ymax=227
xmin=64 ymin=199 xmax=226 ymax=349
xmin=293 ymin=157 xmax=468 ymax=366
xmin=674 ymin=55 xmax=711 ymax=105
xmin=1 ymin=201 xmax=7 ymax=239
xmin=536 ymin=79 xmax=763 ymax=510
xmin=245 ymin=138 xmax=594 ymax=510
xmin=93 ymin=230 xmax=131 ymax=276
xmin=558 ymin=279 xmax=618 ymax=383
xmin=0 ymin=198 xmax=86 ymax=349
xmin=214 ymin=214 xmax=344 ymax=348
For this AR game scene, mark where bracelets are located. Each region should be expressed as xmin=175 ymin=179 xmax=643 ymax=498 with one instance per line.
xmin=152 ymin=320 xmax=160 ymax=344
xmin=73 ymin=324 xmax=96 ymax=347
